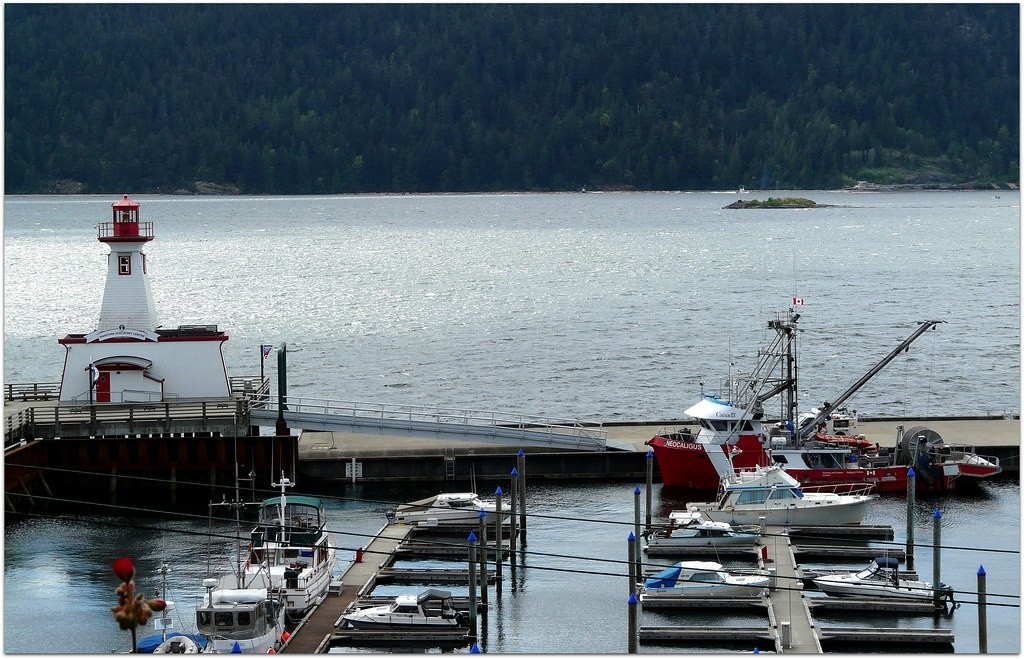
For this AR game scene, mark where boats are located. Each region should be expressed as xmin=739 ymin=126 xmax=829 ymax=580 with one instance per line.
xmin=342 ymin=589 xmax=458 ymax=627
xmin=108 ymin=431 xmax=333 ymax=655
xmin=646 ymin=510 xmax=763 ymax=549
xmin=641 ymin=559 xmax=770 ymax=599
xmin=687 ymin=451 xmax=879 ymax=527
xmin=642 ymin=293 xmax=1004 ymax=490
xmin=810 ymin=557 xmax=948 ymax=601
xmin=385 ymin=493 xmax=512 ymax=527
